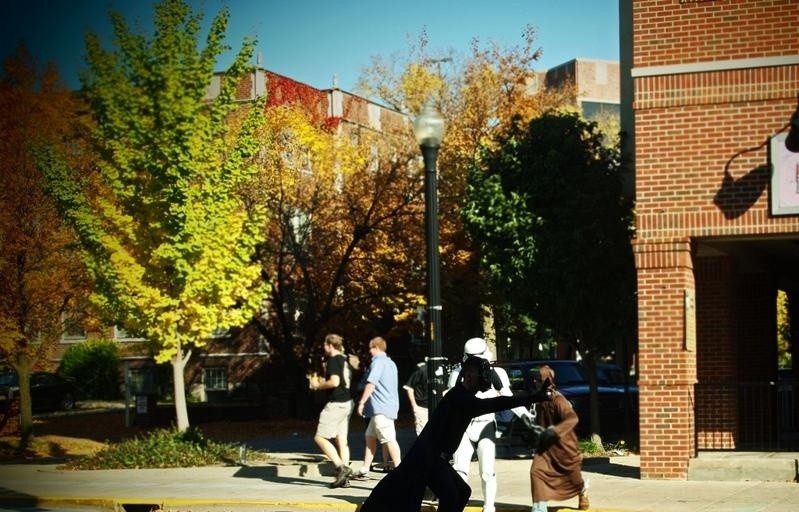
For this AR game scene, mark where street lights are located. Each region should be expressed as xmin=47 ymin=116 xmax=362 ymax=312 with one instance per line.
xmin=410 ymin=89 xmax=447 ymax=424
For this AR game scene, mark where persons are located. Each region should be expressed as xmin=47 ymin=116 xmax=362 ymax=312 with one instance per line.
xmin=307 ymin=333 xmax=354 ymax=487
xmin=528 ymin=365 xmax=589 ymax=512
xmin=403 ymin=357 xmax=438 ymax=440
xmin=360 ymin=354 xmax=550 ymax=511
xmin=356 ymin=337 xmax=405 ymax=476
xmin=441 ymin=336 xmax=535 ymax=512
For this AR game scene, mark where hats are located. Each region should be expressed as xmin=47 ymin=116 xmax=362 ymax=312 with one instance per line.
xmin=462 ymin=337 xmax=491 ymax=360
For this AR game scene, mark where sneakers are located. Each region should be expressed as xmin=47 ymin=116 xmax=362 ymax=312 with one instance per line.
xmin=331 ymin=463 xmax=394 ymax=488
xmin=579 ymin=492 xmax=589 ymax=509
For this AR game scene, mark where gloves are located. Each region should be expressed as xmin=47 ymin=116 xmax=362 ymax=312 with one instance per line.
xmin=529 ymin=379 xmax=550 ymax=401
xmin=540 ymin=430 xmax=556 ymax=450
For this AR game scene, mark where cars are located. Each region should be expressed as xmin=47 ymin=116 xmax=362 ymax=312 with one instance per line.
xmin=493 ymin=357 xmax=639 ymax=461
xmin=1 ymin=370 xmax=80 ymax=413
